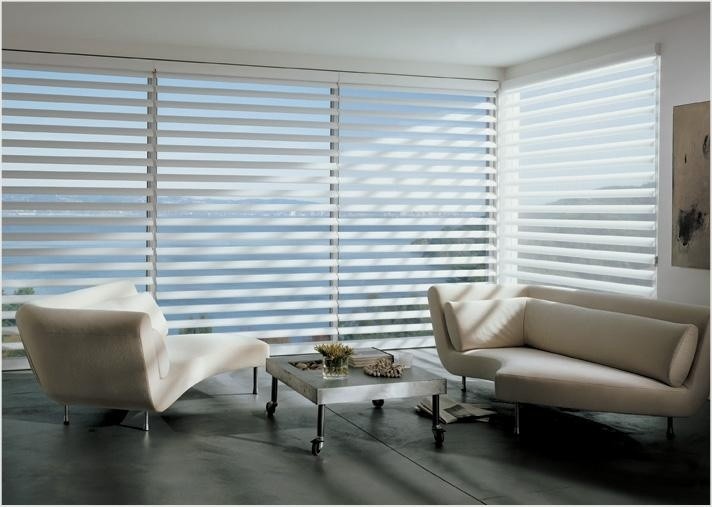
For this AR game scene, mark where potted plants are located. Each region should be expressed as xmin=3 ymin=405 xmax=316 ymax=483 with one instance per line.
xmin=316 ymin=344 xmax=355 ymax=381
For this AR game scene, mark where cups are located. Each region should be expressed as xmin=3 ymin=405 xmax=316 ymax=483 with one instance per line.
xmin=399 ymin=353 xmax=413 ymax=369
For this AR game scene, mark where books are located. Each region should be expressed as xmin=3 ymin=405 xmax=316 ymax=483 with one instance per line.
xmin=349 ymin=346 xmax=395 ymax=369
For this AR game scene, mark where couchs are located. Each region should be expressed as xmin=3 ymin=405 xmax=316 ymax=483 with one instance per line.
xmin=427 ymin=279 xmax=710 ymax=435
xmin=14 ymin=282 xmax=270 ymax=431
